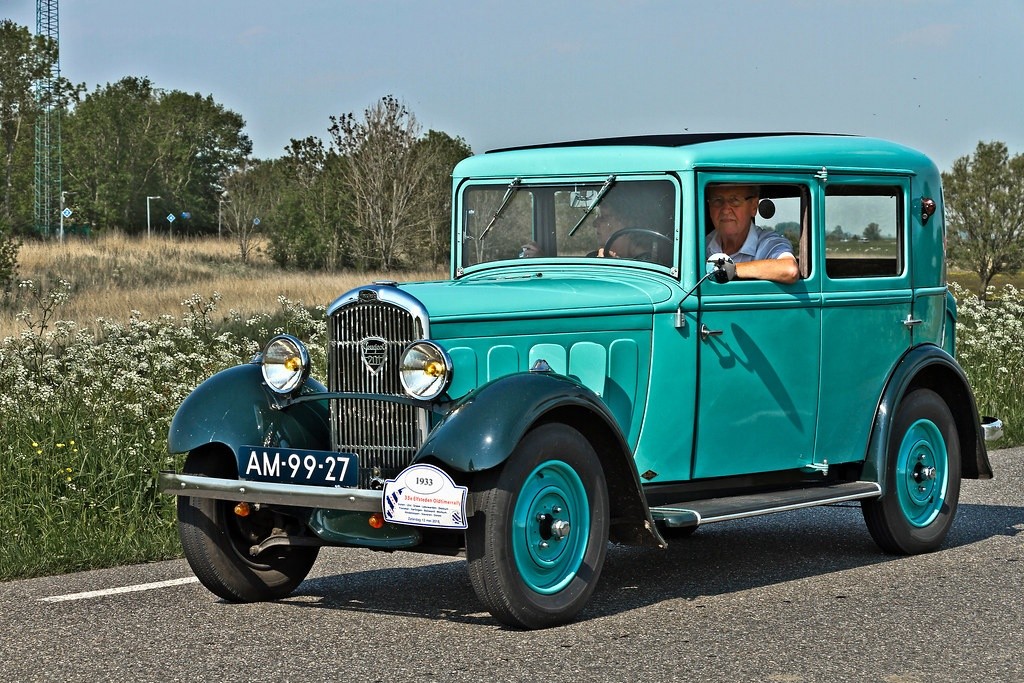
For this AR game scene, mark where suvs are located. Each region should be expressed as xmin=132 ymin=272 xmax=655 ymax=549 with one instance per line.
xmin=154 ymin=133 xmax=1006 ymax=631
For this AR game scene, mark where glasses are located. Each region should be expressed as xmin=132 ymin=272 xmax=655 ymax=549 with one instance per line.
xmin=592 ymin=214 xmax=613 ymax=228
xmin=706 ymin=194 xmax=754 ymax=208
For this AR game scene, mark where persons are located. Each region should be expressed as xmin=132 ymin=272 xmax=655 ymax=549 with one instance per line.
xmin=520 ymin=184 xmax=660 ymax=265
xmin=597 ymin=181 xmax=801 ymax=285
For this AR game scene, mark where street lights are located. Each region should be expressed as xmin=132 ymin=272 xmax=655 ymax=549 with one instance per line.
xmin=146 ymin=195 xmax=161 ymax=240
